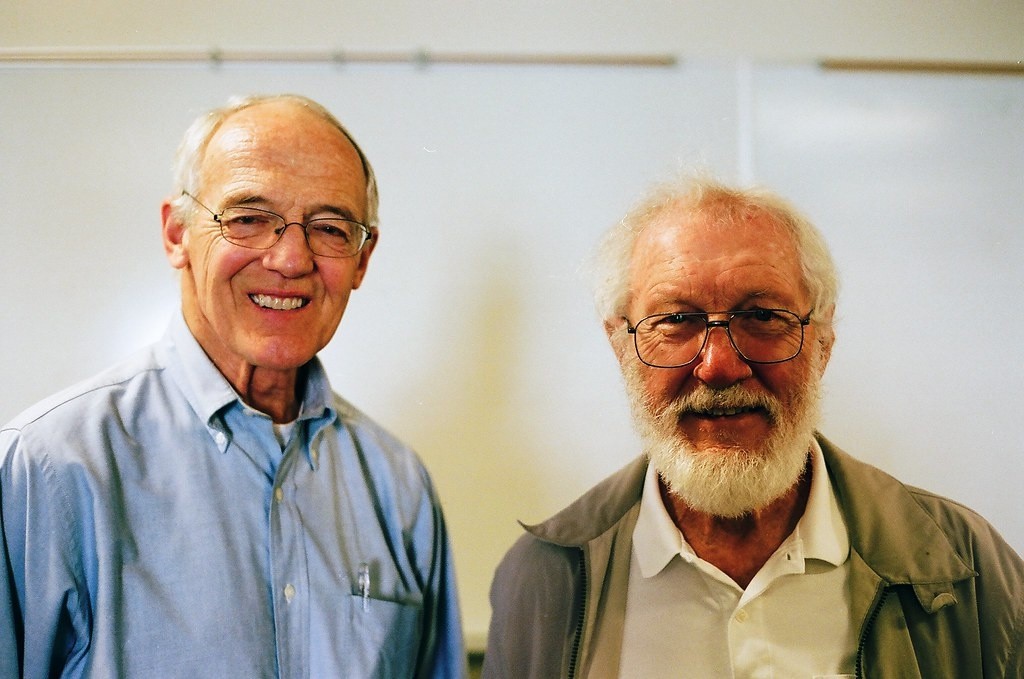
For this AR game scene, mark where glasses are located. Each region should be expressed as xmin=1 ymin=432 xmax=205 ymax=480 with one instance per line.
xmin=622 ymin=308 xmax=815 ymax=369
xmin=182 ymin=190 xmax=373 ymax=259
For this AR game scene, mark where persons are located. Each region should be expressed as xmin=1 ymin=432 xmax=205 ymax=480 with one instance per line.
xmin=481 ymin=170 xmax=1024 ymax=679
xmin=0 ymin=93 xmax=468 ymax=679
